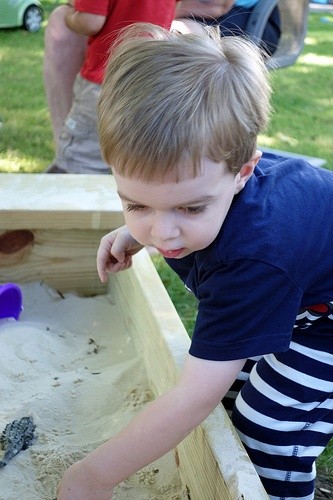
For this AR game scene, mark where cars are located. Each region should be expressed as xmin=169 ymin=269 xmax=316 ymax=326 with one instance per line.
xmin=0 ymin=0 xmax=45 ymax=34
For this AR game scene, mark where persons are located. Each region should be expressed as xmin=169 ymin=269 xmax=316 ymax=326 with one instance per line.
xmin=43 ymin=1 xmax=281 ymax=177
xmin=52 ymin=25 xmax=333 ymax=500
